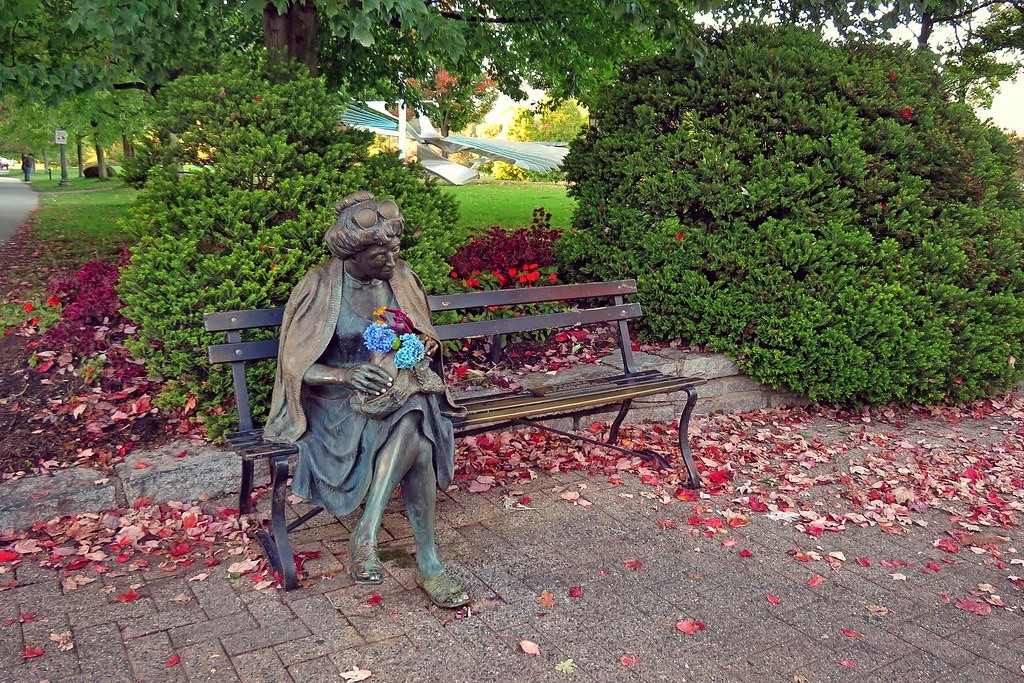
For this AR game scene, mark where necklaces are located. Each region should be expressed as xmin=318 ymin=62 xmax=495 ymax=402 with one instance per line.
xmin=343 ymin=260 xmax=370 ymax=285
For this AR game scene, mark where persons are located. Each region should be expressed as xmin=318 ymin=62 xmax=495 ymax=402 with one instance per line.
xmin=263 ymin=190 xmax=470 ymax=607
xmin=0 ymin=161 xmax=9 ymax=171
xmin=21 ymin=151 xmax=34 ymax=182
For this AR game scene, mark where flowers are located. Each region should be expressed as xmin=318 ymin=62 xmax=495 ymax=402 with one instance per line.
xmin=362 ymin=306 xmax=427 ymax=372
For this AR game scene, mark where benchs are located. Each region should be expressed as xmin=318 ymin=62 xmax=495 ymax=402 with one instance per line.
xmin=203 ymin=275 xmax=707 ymax=594
